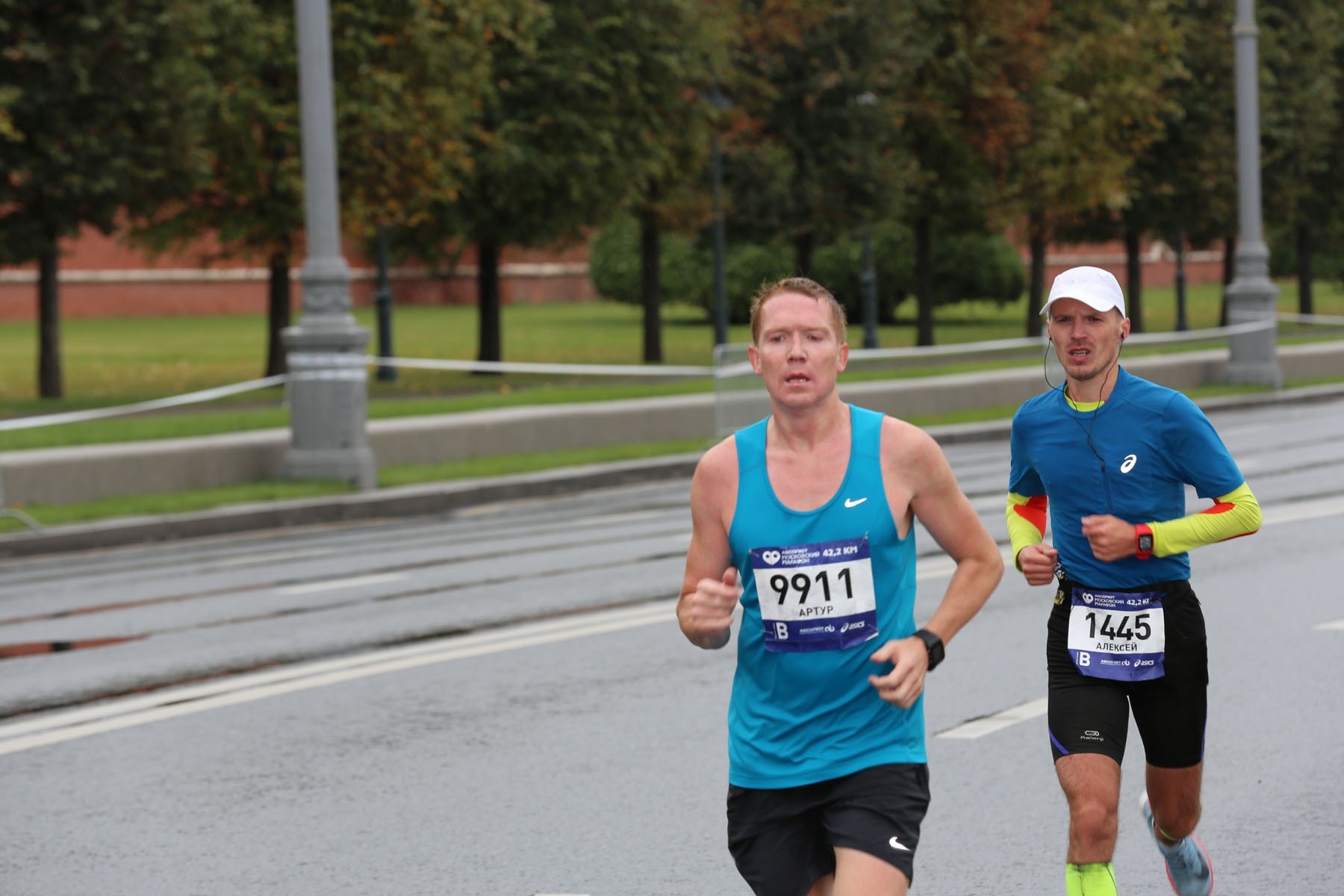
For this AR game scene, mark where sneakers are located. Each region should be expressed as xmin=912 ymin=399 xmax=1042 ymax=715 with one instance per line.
xmin=1139 ymin=799 xmax=1214 ymax=896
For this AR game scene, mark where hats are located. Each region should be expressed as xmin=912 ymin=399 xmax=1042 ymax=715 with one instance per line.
xmin=1039 ymin=266 xmax=1127 ymax=321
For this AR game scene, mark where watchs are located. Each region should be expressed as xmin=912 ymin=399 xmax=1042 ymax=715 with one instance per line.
xmin=1133 ymin=524 xmax=1155 ymax=560
xmin=911 ymin=627 xmax=947 ymax=672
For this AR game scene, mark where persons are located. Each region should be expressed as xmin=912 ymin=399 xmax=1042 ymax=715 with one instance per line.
xmin=1017 ymin=267 xmax=1264 ymax=896
xmin=675 ymin=277 xmax=1003 ymax=896
